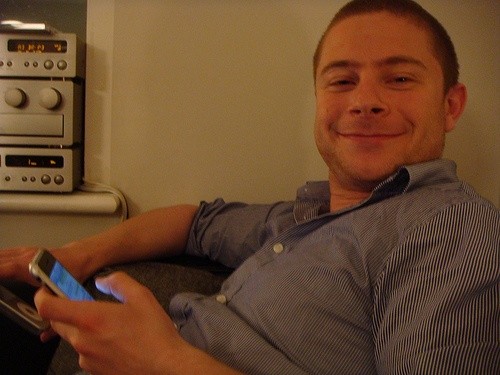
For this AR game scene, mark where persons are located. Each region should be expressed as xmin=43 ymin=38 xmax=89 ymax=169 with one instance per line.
xmin=0 ymin=0 xmax=500 ymax=375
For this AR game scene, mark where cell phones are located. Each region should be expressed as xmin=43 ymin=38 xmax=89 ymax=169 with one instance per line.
xmin=28 ymin=248 xmax=94 ymax=302
